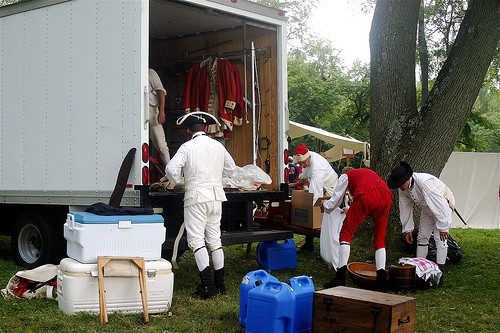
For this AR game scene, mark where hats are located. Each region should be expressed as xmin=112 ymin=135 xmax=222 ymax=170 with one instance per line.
xmin=176 ymin=110 xmax=219 ymax=126
xmin=387 ymin=161 xmax=413 ymax=189
xmin=293 ymin=143 xmax=311 ymax=162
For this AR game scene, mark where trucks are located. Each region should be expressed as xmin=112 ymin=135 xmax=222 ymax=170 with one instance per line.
xmin=0 ymin=0 xmax=295 ymax=268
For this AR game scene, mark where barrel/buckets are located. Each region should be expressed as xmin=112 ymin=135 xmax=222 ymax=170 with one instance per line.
xmin=239 ymin=269 xmax=315 ymax=333
xmin=389 ymin=264 xmax=431 ymax=292
xmin=257 ymin=239 xmax=296 ymax=272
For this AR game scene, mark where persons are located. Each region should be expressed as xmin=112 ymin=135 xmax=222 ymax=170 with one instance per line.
xmin=166 ymin=112 xmax=235 ymax=299
xmin=149 ymin=68 xmax=170 ymax=166
xmin=320 ymin=167 xmax=393 ymax=292
xmin=387 ymin=161 xmax=455 ymax=276
xmin=289 ymin=143 xmax=339 ymax=255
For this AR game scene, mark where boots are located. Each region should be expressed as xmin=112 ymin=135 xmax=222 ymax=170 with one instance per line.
xmin=431 ymin=264 xmax=444 ymax=288
xmin=374 ymin=269 xmax=388 ymax=293
xmin=192 ymin=266 xmax=227 ymax=299
xmin=323 ymin=265 xmax=347 ymax=288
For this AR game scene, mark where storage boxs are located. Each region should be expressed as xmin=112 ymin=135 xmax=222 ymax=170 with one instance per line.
xmin=293 ymin=189 xmax=332 ymax=229
xmin=62 ymin=211 xmax=165 ymax=263
xmin=57 ymin=259 xmax=175 ymax=315
xmin=311 ymin=285 xmax=416 ymax=333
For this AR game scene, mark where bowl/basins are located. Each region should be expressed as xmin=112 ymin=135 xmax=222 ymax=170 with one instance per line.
xmin=346 ymin=262 xmax=389 ymax=290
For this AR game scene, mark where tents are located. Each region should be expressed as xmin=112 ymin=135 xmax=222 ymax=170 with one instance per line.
xmin=289 ymin=121 xmax=370 ymax=162
xmin=438 ymin=152 xmax=500 ymax=231
